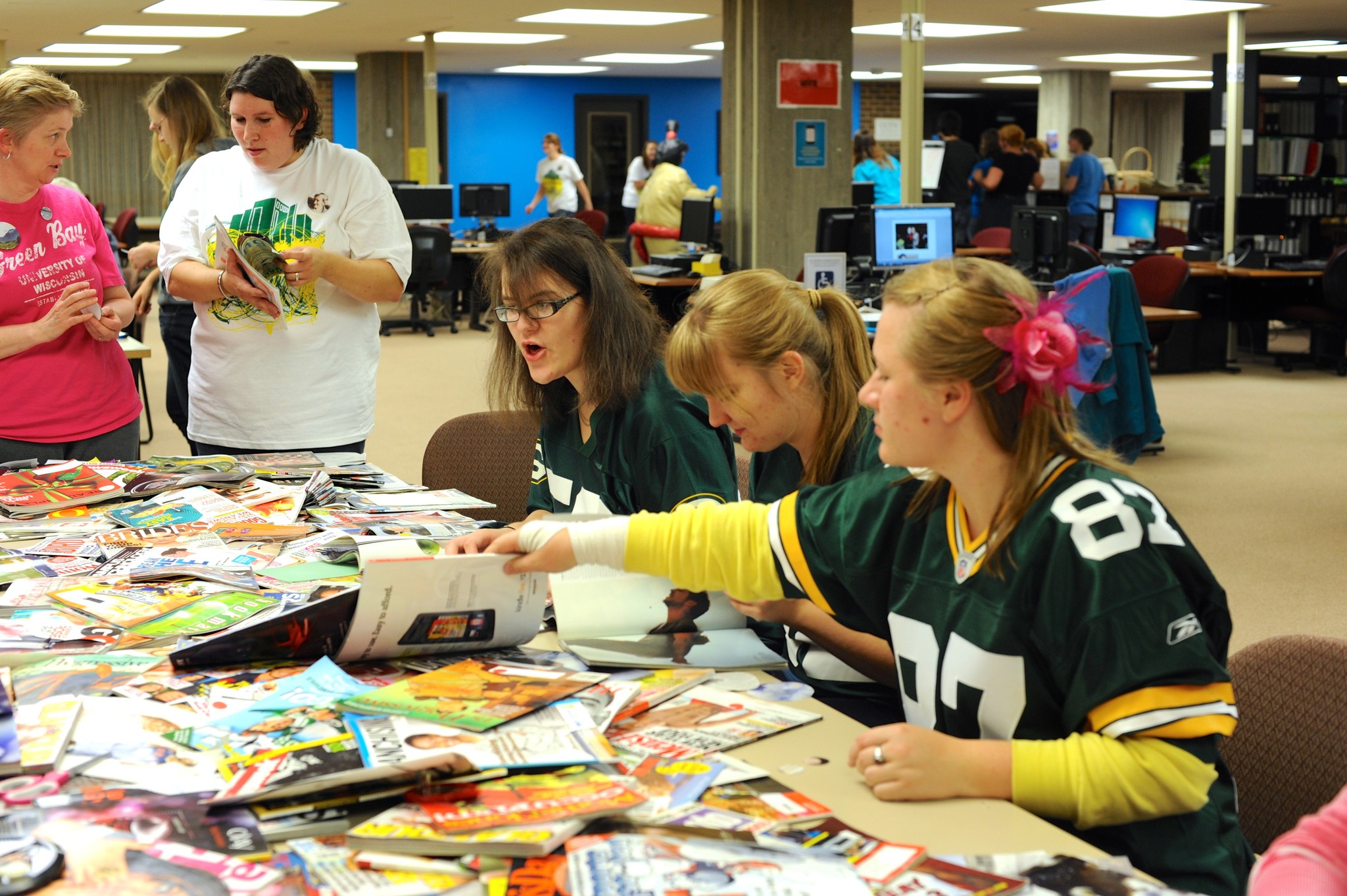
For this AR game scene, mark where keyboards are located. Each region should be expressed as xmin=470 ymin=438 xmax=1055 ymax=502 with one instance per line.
xmin=633 ymin=263 xmax=683 ymax=277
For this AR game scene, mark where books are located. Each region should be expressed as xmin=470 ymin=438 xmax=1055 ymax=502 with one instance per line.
xmin=212 ymin=216 xmax=289 ymax=332
xmin=0 ymin=450 xmax=1204 ymax=896
xmin=1254 ymin=99 xmax=1347 ymax=254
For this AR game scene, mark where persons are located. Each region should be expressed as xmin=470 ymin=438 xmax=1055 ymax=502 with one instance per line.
xmin=156 ymin=55 xmax=412 ymax=457
xmin=0 ymin=64 xmax=143 ymax=468
xmin=850 ymin=116 xmax=1107 ymax=249
xmin=574 ymin=682 xmax=611 ymax=726
xmin=128 ymin=75 xmax=239 ymax=455
xmin=526 ymin=134 xmax=593 ymax=219
xmin=668 ymin=267 xmax=906 ymax=730
xmin=650 ymin=587 xmax=710 ymax=635
xmin=619 ymin=139 xmax=726 ymax=268
xmin=405 ymin=717 xmax=571 ymax=758
xmin=444 ymin=216 xmax=740 ymax=557
xmin=1248 ymin=781 xmax=1347 ymax=896
xmin=486 ymin=257 xmax=1260 ymax=896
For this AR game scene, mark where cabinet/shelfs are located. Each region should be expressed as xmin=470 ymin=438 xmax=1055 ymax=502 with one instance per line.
xmin=1209 ymin=49 xmax=1347 ymax=200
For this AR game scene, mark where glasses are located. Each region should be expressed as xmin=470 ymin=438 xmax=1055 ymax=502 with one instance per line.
xmin=494 ymin=285 xmax=587 ymax=322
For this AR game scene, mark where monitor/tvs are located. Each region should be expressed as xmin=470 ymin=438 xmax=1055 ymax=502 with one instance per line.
xmin=815 ymin=182 xmax=1292 ymax=274
xmin=460 ymin=184 xmax=510 ymax=231
xmin=391 ymin=185 xmax=453 ymax=220
xmin=677 ymin=199 xmax=712 ymax=257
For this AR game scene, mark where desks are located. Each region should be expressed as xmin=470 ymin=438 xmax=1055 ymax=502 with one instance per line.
xmin=0 ymin=452 xmax=1177 ymax=896
xmin=1187 ymin=263 xmax=1323 ymax=277
xmin=629 ymin=266 xmax=700 ymax=286
xmin=1142 ymin=305 xmax=1200 ymax=320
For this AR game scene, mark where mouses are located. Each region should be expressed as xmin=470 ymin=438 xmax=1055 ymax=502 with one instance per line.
xmin=686 ymin=271 xmax=704 ymax=278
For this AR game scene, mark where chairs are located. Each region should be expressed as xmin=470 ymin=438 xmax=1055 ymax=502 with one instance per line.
xmin=972 ymin=227 xmax=1011 ymax=248
xmin=380 ymin=225 xmax=456 ymax=336
xmin=423 ymin=410 xmax=540 ymax=522
xmin=1218 ymin=634 xmax=1347 ymax=855
xmin=1157 ymin=224 xmax=1189 ymax=248
xmin=1128 ymin=255 xmax=1190 ymax=342
xmin=1275 ymin=245 xmax=1347 ymax=375
xmin=113 ymin=208 xmax=138 ymax=245
xmin=572 ymin=210 xmax=608 ymax=242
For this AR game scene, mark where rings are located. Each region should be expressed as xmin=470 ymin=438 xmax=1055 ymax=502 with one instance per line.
xmin=874 ymin=747 xmax=885 ymax=763
xmin=99 ymin=335 xmax=104 ymax=339
xmin=295 ymin=272 xmax=299 ymax=281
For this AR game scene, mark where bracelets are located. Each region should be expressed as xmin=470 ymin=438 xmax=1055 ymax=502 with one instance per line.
xmin=218 ymin=271 xmax=238 ymax=300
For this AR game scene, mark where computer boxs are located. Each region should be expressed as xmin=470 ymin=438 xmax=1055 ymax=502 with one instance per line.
xmin=650 ymin=254 xmax=730 ymax=272
xmin=1098 ymin=249 xmax=1175 ymax=268
xmin=1183 ymin=246 xmax=1225 ymax=261
xmin=1234 ymin=249 xmax=1302 ymax=268
xmin=463 ymin=230 xmax=510 ymax=242
